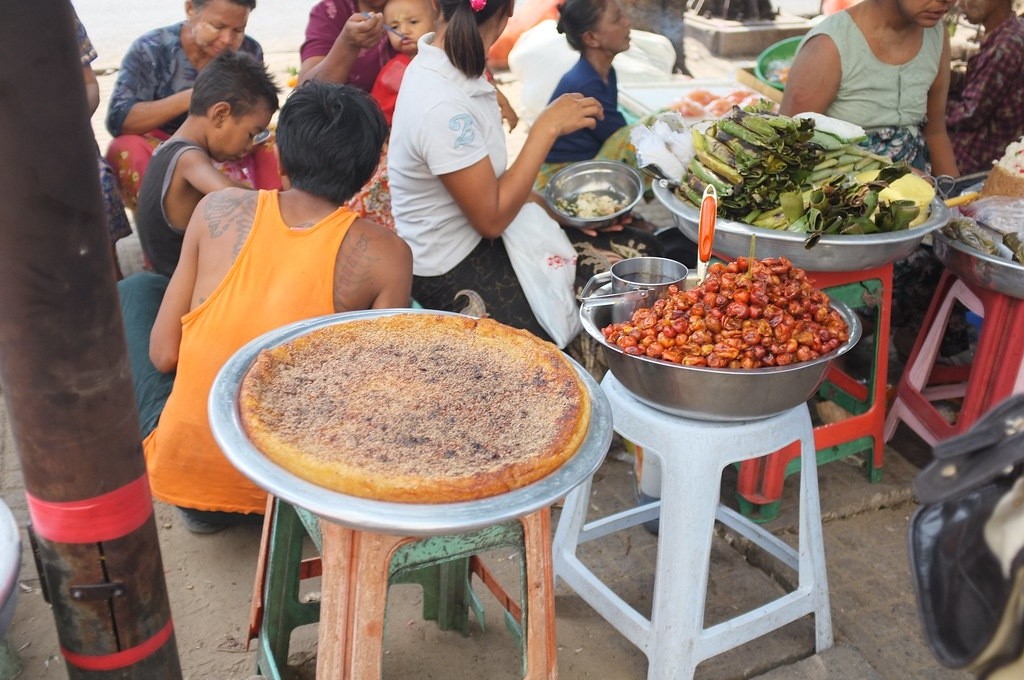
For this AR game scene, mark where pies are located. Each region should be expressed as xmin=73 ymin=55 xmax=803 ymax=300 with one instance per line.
xmin=240 ymin=313 xmax=591 ymax=504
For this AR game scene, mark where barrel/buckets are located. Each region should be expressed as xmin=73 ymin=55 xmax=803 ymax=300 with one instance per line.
xmin=581 ymin=257 xmax=688 ymax=323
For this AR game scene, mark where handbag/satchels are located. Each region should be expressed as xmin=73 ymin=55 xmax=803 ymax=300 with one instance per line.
xmin=498 ymin=204 xmax=584 ymax=348
xmin=908 ymin=394 xmax=1024 ymax=677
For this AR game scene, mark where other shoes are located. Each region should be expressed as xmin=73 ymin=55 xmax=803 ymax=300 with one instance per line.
xmin=178 ymin=508 xmax=224 ymax=534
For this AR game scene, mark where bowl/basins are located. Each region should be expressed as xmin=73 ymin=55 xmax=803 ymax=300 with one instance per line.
xmin=543 ymin=159 xmax=643 ymax=230
xmin=579 ymin=273 xmax=862 ymax=422
xmin=651 ymin=174 xmax=950 ymax=272
xmin=931 ymin=173 xmax=1024 ymax=299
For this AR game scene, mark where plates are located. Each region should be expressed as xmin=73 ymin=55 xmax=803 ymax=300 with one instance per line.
xmin=205 ymin=307 xmax=615 ymax=533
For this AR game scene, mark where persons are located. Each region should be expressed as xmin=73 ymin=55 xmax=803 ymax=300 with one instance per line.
xmin=106 ymin=0 xmax=284 ymax=275
xmin=135 ymin=49 xmax=285 ymax=279
xmin=142 ymin=71 xmax=413 ymax=535
xmin=298 ymin=0 xmax=401 ymax=94
xmin=69 ymin=1 xmax=134 ymax=280
xmin=778 ymin=0 xmax=973 ymax=388
xmin=370 ymin=0 xmax=521 ymax=135
xmin=388 ymin=0 xmax=606 ymax=358
xmin=532 ymin=0 xmax=657 ymax=204
xmin=945 ymin=0 xmax=1024 ymax=179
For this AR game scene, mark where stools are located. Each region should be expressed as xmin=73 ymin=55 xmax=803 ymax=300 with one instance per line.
xmin=247 ymin=250 xmax=1024 ymax=680
